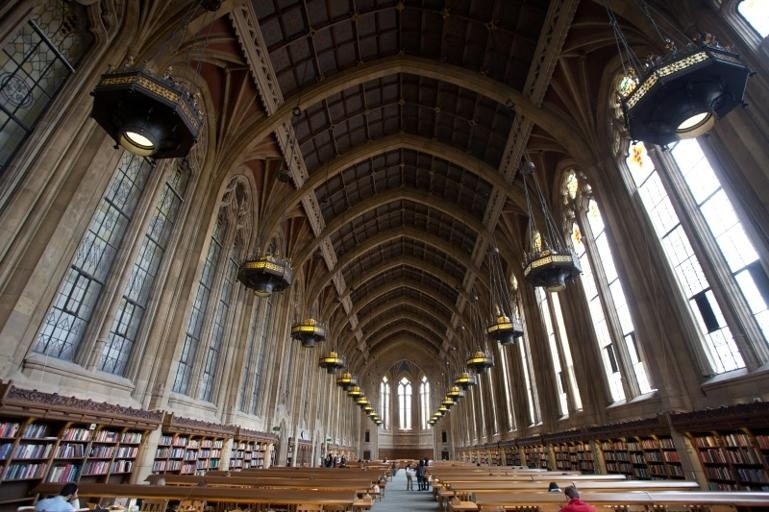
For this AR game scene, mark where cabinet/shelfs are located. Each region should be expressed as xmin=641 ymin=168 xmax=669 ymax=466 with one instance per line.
xmin=0 ymin=402 xmax=357 ymax=512
xmin=459 ymin=433 xmax=769 ymax=492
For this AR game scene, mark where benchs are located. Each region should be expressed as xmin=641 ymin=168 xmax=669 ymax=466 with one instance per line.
xmin=423 ymin=455 xmax=769 ymax=512
xmin=17 ymin=460 xmax=395 ymax=512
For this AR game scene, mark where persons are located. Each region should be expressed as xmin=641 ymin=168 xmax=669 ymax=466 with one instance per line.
xmin=549 ymin=482 xmax=561 ymax=492
xmin=558 ymin=485 xmax=597 ymax=512
xmin=323 ymin=451 xmax=432 ymax=491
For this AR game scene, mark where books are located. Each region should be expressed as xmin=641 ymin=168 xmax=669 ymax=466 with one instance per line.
xmin=695 ymin=435 xmax=768 ymax=492
xmin=0 ymin=411 xmax=284 ymax=512
xmin=505 ymin=443 xmax=549 ymax=470
xmin=459 ymin=447 xmax=501 ymax=467
xmin=554 ymin=442 xmax=596 ymax=475
xmin=601 ymin=439 xmax=684 ymax=481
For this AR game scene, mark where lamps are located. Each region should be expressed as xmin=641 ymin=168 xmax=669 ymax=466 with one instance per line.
xmin=499 ymin=95 xmax=583 ymax=292
xmin=596 ymin=4 xmax=758 ymax=153
xmin=427 ymin=231 xmax=524 ymax=425
xmin=236 ymin=38 xmax=314 ymax=298
xmin=290 ymin=208 xmax=388 ymax=427
xmin=88 ymin=0 xmax=208 ymax=160
